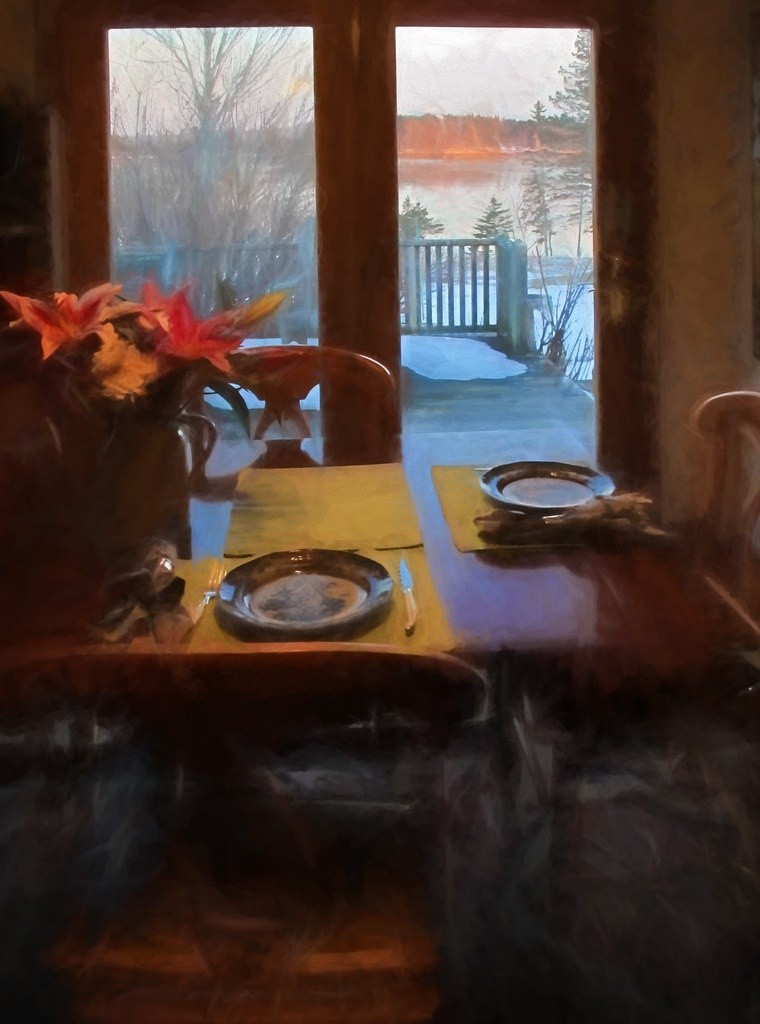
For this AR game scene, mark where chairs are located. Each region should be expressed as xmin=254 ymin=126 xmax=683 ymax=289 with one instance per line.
xmin=186 ymin=346 xmax=399 ymax=463
xmin=667 ymin=391 xmax=760 ymax=625
xmin=168 ymin=647 xmax=490 ymax=1006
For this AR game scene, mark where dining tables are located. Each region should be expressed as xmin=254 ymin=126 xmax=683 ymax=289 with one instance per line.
xmin=0 ymin=424 xmax=723 ymax=704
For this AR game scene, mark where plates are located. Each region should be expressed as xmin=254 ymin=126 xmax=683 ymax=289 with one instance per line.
xmin=479 ymin=461 xmax=614 ymax=507
xmin=217 ymin=549 xmax=392 ymax=629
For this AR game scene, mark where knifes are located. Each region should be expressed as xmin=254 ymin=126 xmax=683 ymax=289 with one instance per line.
xmin=399 ymin=558 xmax=416 ymax=631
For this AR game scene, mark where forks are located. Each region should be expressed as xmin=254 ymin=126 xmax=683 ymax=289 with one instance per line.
xmin=192 ymin=569 xmax=228 ymax=625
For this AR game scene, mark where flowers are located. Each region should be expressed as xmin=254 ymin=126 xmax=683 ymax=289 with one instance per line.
xmin=0 ymin=276 xmax=285 ymax=417
